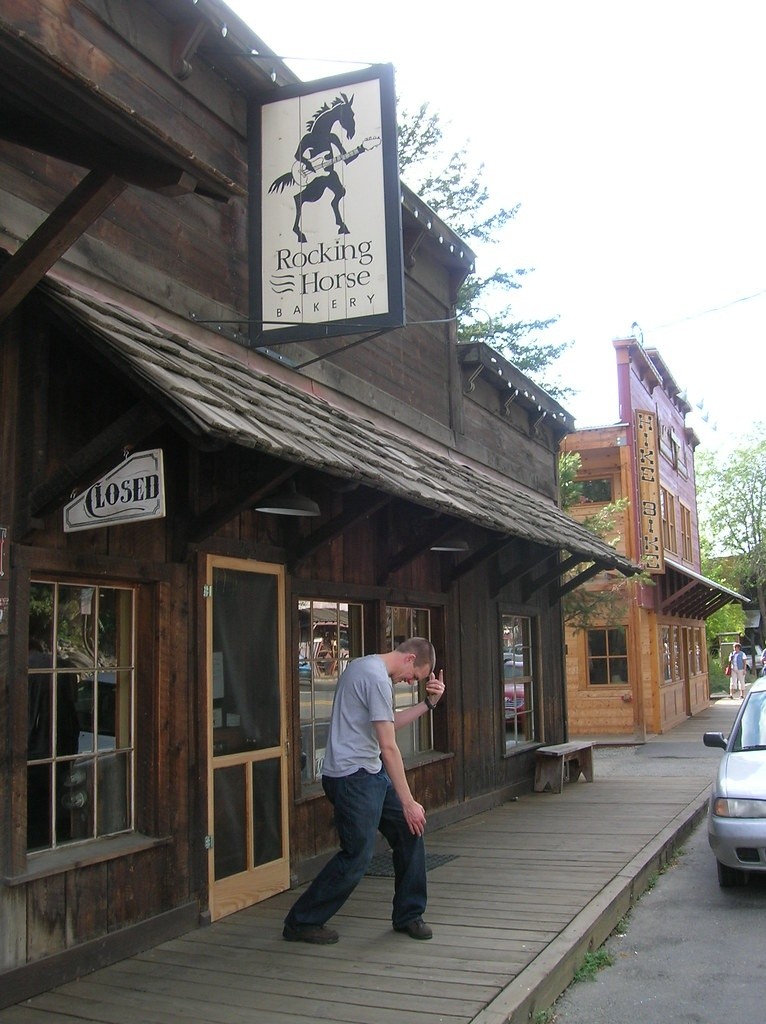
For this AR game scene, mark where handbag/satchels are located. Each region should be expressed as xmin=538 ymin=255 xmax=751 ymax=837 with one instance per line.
xmin=725 ymin=665 xmax=731 ymax=675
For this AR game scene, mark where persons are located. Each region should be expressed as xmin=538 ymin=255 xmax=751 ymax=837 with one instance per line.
xmin=728 ymin=643 xmax=747 ymax=699
xmin=282 ymin=638 xmax=445 ymax=942
xmin=27 ymin=639 xmax=80 ymax=853
xmin=761 ymin=648 xmax=766 ymax=677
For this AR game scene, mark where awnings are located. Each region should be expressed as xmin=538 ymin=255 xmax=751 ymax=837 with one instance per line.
xmin=300 ymin=606 xmax=349 ymax=630
xmin=650 ymin=553 xmax=751 ymax=619
xmin=0 ymin=236 xmax=640 ymax=613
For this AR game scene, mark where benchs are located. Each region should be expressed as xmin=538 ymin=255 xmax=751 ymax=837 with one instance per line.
xmin=535 ymin=740 xmax=599 ymax=794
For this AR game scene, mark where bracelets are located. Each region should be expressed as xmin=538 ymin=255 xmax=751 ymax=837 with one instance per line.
xmin=425 ymin=695 xmax=437 ymax=709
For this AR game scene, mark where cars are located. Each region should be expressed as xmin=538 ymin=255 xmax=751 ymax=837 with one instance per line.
xmin=76 ymin=670 xmax=117 ymax=753
xmin=299 ymin=653 xmax=312 ymax=685
xmin=740 ymin=645 xmax=763 ymax=673
xmin=502 ymin=651 xmax=524 ymax=724
xmin=702 ymin=674 xmax=766 ymax=887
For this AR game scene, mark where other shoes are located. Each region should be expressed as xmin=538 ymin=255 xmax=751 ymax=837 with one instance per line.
xmin=739 ymin=696 xmax=743 ymax=699
xmin=392 ymin=916 xmax=434 ymax=941
xmin=281 ymin=924 xmax=340 ymax=945
xmin=727 ymin=695 xmax=733 ymax=699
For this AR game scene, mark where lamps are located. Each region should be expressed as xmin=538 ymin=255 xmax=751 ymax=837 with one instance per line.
xmin=429 ymin=539 xmax=470 ymax=552
xmin=252 ymin=475 xmax=322 ymax=517
